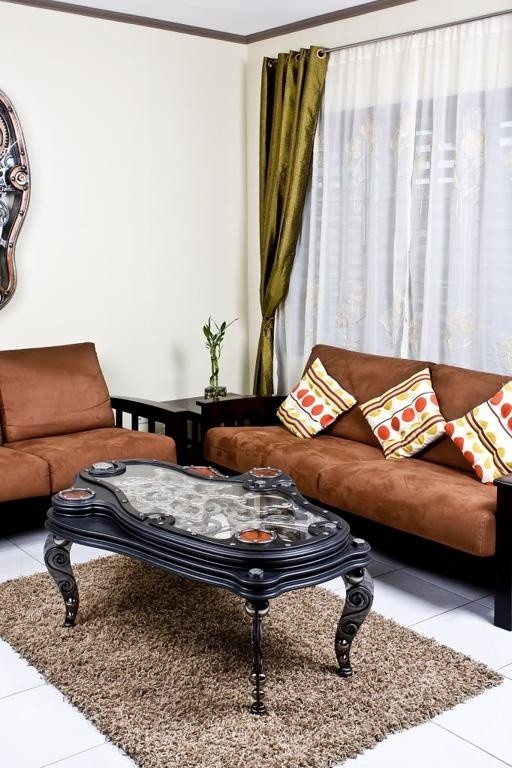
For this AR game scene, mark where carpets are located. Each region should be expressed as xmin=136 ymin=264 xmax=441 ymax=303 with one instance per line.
xmin=2 ymin=549 xmax=505 ymax=767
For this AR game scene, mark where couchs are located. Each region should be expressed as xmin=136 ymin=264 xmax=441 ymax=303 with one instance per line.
xmin=0 ymin=340 xmax=181 ymax=549
xmin=196 ymin=344 xmax=512 ymax=632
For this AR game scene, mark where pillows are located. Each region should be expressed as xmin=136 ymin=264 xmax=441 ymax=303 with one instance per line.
xmin=277 ymin=357 xmax=357 ymax=438
xmin=356 ymin=366 xmax=449 ymax=461
xmin=442 ymin=381 xmax=512 ymax=485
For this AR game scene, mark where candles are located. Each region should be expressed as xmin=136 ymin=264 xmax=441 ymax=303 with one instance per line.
xmin=201 ymin=314 xmax=239 ymax=400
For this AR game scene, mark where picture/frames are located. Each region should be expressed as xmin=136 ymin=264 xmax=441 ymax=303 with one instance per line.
xmin=0 ymin=90 xmax=33 ymax=308
